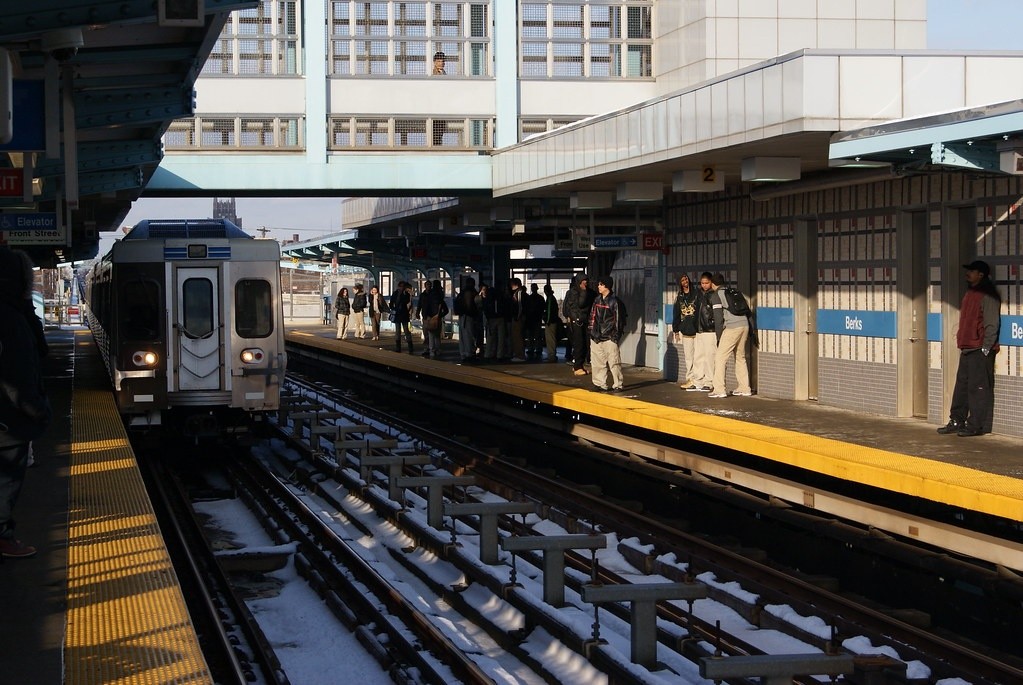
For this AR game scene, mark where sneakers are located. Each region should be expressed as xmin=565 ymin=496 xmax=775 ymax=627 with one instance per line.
xmin=957 ymin=425 xmax=981 ymax=436
xmin=936 ymin=419 xmax=967 ymax=435
xmin=680 ymin=380 xmax=696 ymax=389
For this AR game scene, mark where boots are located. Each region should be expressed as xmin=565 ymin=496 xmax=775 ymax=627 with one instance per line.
xmin=394 ymin=339 xmax=402 ymax=352
xmin=407 ymin=340 xmax=413 ymax=354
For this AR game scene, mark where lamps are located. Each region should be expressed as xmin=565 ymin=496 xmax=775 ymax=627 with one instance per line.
xmin=511 ymin=198 xmax=525 ymax=236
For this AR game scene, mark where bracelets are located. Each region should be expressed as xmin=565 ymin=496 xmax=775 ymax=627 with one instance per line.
xmin=675 ymin=334 xmax=678 ymax=336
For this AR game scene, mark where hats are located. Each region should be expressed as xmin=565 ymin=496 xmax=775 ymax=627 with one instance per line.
xmin=963 ymin=261 xmax=990 ymax=275
xmin=598 ymin=276 xmax=613 ymax=288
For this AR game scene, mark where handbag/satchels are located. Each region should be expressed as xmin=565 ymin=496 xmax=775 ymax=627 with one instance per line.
xmin=427 ymin=314 xmax=438 ymax=330
xmin=388 ymin=310 xmax=399 ymax=322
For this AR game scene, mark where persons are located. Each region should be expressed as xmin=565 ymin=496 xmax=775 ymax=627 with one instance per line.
xmin=937 ymin=260 xmax=1001 ymax=437
xmin=588 ymin=276 xmax=624 ymax=393
xmin=458 ymin=277 xmax=529 ymax=365
xmin=562 ymin=273 xmax=599 ymax=375
xmin=335 ymin=287 xmax=350 ymax=340
xmin=389 ymin=281 xmax=414 ymax=355
xmin=523 ymin=283 xmax=545 ymax=362
xmin=672 ymin=271 xmax=759 ymax=398
xmin=416 ymin=280 xmax=449 ymax=360
xmin=352 ymin=285 xmax=367 ymax=339
xmin=0 ymin=243 xmax=57 ymax=556
xmin=542 ymin=284 xmax=559 ymax=362
xmin=368 ymin=285 xmax=390 ymax=341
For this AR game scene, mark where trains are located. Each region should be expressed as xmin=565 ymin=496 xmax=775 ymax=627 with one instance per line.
xmin=83 ymin=217 xmax=290 ymax=444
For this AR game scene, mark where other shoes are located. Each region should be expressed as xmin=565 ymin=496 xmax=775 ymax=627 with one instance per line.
xmin=574 ymin=369 xmax=586 ymax=376
xmin=708 ymin=392 xmax=727 ymax=398
xmin=420 ymin=349 xmax=508 ymax=364
xmin=686 ymin=385 xmax=698 ymax=391
xmin=730 ymin=390 xmax=750 ymax=397
xmin=589 ymin=385 xmax=602 ymax=392
xmin=700 ymin=386 xmax=711 ymax=391
xmin=614 ymin=388 xmax=622 ymax=392
xmin=510 ymin=357 xmax=525 ymax=361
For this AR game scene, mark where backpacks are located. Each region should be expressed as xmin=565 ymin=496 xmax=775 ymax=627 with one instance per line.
xmin=716 ymin=284 xmax=750 ymax=316
xmin=454 ymin=291 xmax=464 ymax=315
